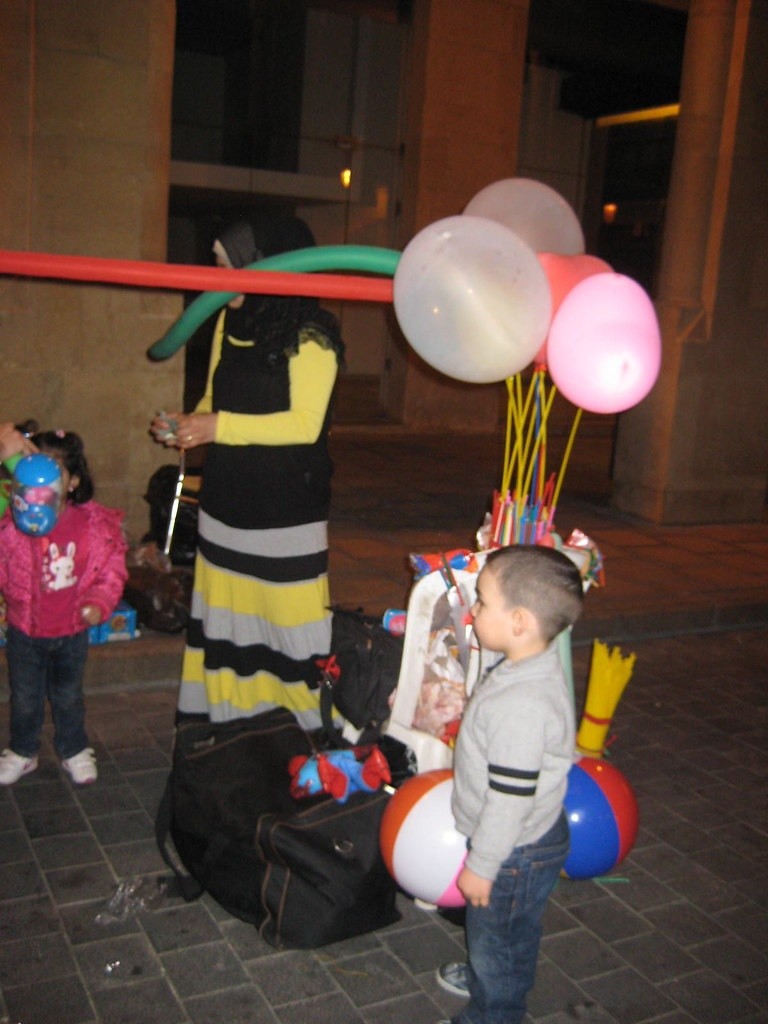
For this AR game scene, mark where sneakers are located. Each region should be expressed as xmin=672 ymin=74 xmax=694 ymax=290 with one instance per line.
xmin=1 ymin=747 xmax=39 ymax=785
xmin=435 ymin=961 xmax=470 ymax=998
xmin=63 ymin=747 xmax=98 ymax=786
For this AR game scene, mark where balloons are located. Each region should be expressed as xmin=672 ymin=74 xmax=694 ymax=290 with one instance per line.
xmin=391 ymin=215 xmax=552 ymax=384
xmin=547 ymin=271 xmax=662 ymax=414
xmin=463 ymin=176 xmax=585 ymax=256
xmin=530 ymin=253 xmax=618 ymax=368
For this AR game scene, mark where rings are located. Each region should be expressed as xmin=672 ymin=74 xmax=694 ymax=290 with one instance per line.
xmin=187 ymin=436 xmax=192 ymax=441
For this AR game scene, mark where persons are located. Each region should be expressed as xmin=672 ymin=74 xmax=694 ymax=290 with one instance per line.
xmin=150 ymin=207 xmax=358 ymax=751
xmin=0 ymin=420 xmax=129 ymax=785
xmin=436 ymin=543 xmax=585 ymax=1024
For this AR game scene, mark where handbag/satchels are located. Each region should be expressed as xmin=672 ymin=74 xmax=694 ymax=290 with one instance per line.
xmin=153 ymin=705 xmax=405 ymax=949
xmin=326 ymin=608 xmax=404 ymax=731
